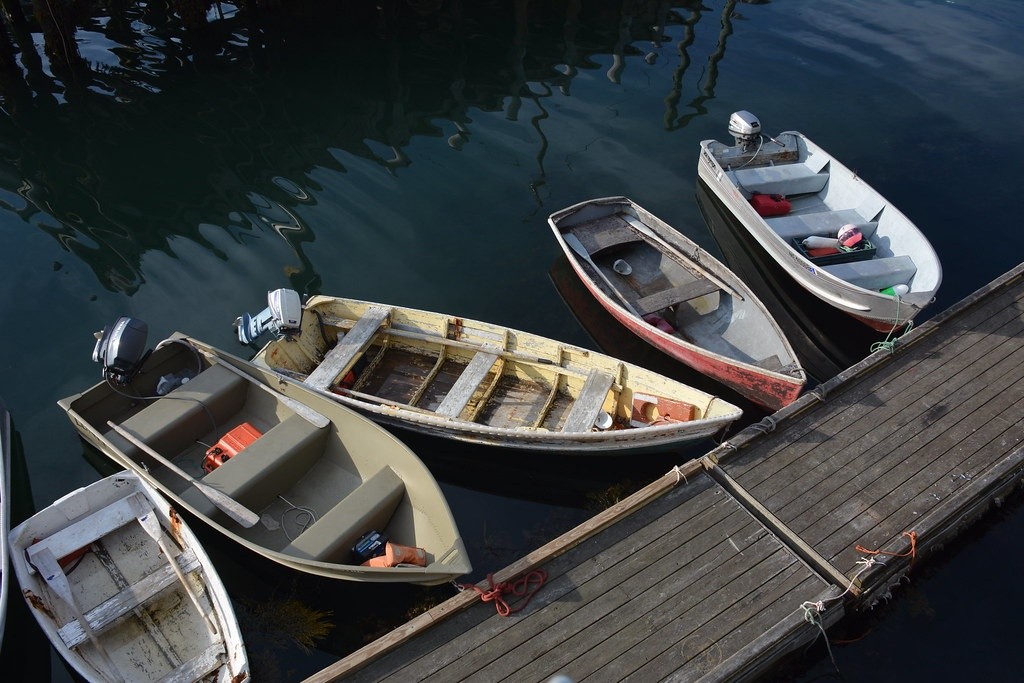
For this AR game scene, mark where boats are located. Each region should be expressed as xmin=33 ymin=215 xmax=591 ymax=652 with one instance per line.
xmin=232 ymin=294 xmax=742 ymax=456
xmin=693 ymin=176 xmax=888 ymax=384
xmin=547 ymin=195 xmax=808 ymax=412
xmin=7 ymin=467 xmax=251 ymax=683
xmin=546 ymin=248 xmax=651 ymax=357
xmin=57 ymin=332 xmax=476 ymax=587
xmin=693 ymin=110 xmax=943 ymax=332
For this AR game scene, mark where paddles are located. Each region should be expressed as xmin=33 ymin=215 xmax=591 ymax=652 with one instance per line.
xmin=124 ymin=490 xmax=219 ymax=635
xmin=620 ymin=211 xmax=746 ymax=302
xmin=27 ymin=545 xmax=126 ymax=683
xmin=562 ymin=231 xmax=646 ymax=324
xmin=105 ymin=417 xmax=262 ymax=530
xmin=197 ymin=346 xmax=332 ymax=431
